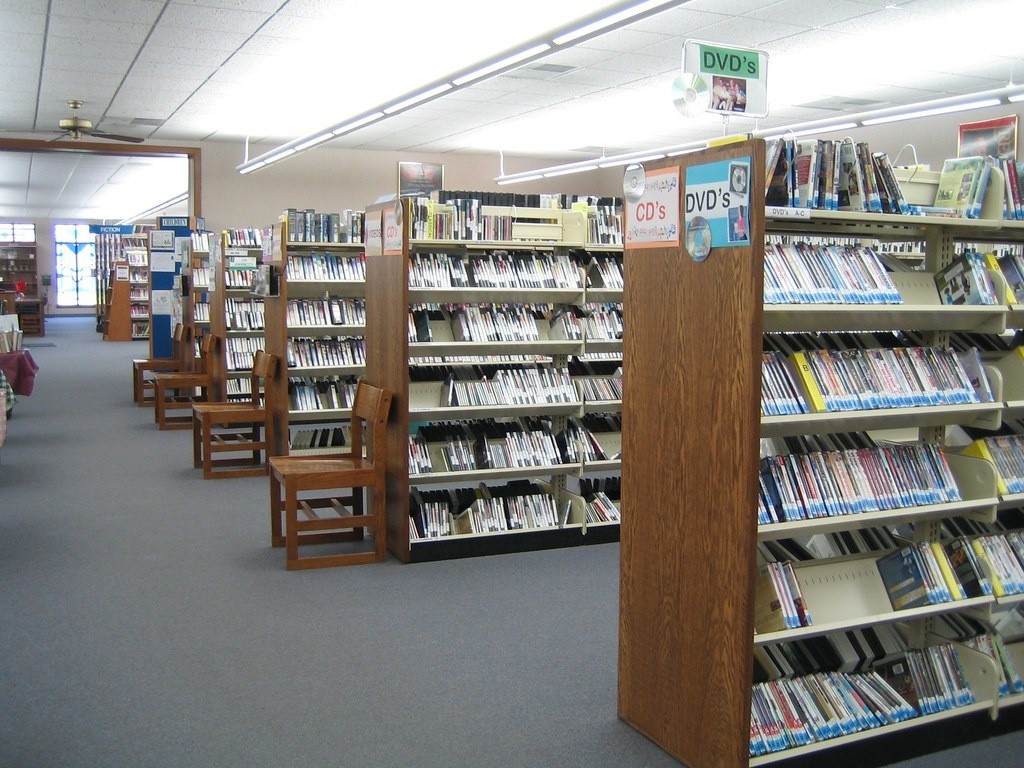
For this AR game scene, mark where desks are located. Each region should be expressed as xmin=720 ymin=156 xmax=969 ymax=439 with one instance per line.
xmin=0 ymin=349 xmax=40 ymax=419
xmin=0 ymin=290 xmax=18 ymax=315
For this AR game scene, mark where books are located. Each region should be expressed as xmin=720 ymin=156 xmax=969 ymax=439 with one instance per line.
xmin=409 ymin=189 xmax=624 ymax=540
xmin=191 ymin=232 xmax=215 ymax=358
xmin=96 ymin=238 xmax=150 ymax=338
xmin=0 ymin=329 xmax=23 ymax=353
xmin=749 ymin=136 xmax=1024 ymax=758
xmin=222 ymin=226 xmax=273 ymax=402
xmin=284 ymin=208 xmax=365 ymax=449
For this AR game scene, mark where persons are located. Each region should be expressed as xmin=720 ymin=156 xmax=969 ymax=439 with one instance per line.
xmin=713 ymin=78 xmax=746 ymax=112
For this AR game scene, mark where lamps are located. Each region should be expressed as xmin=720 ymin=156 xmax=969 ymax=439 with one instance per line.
xmin=235 ymin=0 xmax=691 ymax=176
xmin=495 ymin=66 xmax=1024 ymax=187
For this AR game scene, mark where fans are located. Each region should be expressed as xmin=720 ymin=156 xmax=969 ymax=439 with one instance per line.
xmin=0 ymin=99 xmax=145 ymax=144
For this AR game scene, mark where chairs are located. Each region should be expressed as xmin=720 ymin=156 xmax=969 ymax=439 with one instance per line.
xmin=19 ymin=299 xmax=44 ymax=336
xmin=132 ymin=323 xmax=188 ymax=408
xmin=267 ymin=378 xmax=393 ymax=572
xmin=191 ymin=349 xmax=279 ymax=480
xmin=152 ymin=333 xmax=217 ymax=430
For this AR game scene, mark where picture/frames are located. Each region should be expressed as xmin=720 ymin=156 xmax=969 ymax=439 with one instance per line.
xmin=398 ymin=161 xmax=445 ymax=198
xmin=958 ymin=114 xmax=1019 ymax=161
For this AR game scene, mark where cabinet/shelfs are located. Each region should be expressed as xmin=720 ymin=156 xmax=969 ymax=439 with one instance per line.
xmin=95 ymin=231 xmax=265 ymax=429
xmin=616 ymin=136 xmax=1023 ymax=768
xmin=365 ymin=198 xmax=625 ymax=564
xmin=0 ymin=246 xmax=44 ymax=335
xmin=261 ymin=222 xmax=366 ymax=475
